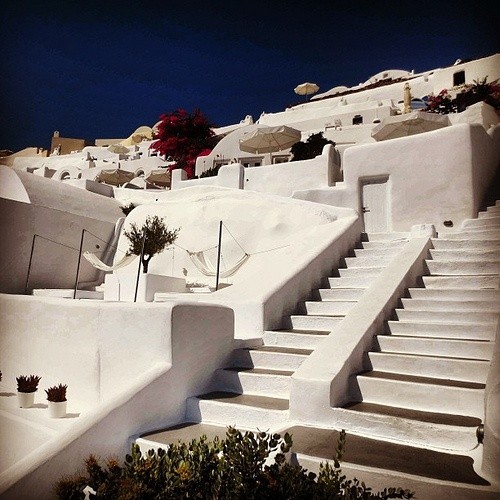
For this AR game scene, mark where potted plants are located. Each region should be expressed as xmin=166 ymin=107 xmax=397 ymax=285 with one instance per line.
xmin=44 ymin=384 xmax=67 ymax=418
xmin=103 ymin=215 xmax=187 ymax=303
xmin=16 ymin=374 xmax=43 ymax=408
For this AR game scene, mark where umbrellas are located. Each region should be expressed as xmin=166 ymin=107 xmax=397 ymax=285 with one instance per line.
xmin=238 ymin=125 xmax=302 ymax=164
xmin=295 ymin=83 xmax=321 ymax=102
